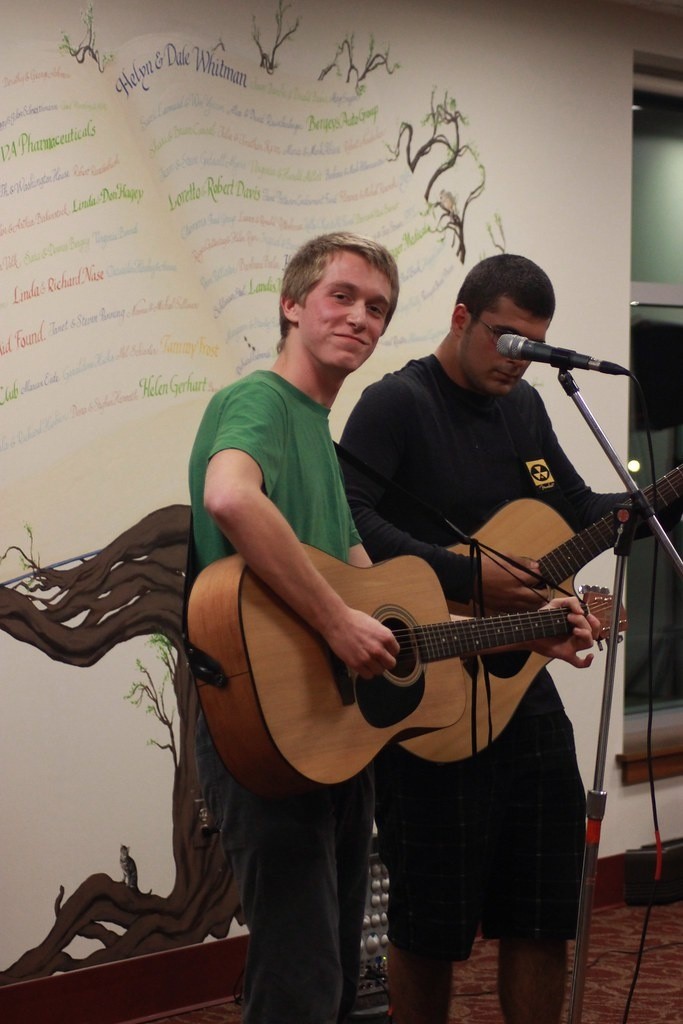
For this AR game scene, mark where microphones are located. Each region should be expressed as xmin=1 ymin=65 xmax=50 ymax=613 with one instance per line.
xmin=495 ymin=334 xmax=630 ymax=376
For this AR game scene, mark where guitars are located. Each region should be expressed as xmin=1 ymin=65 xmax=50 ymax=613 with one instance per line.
xmin=185 ymin=541 xmax=628 ymax=801
xmin=395 ymin=458 xmax=683 ymax=764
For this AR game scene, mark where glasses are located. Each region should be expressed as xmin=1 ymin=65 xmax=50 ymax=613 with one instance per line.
xmin=472 ymin=314 xmax=545 ymax=347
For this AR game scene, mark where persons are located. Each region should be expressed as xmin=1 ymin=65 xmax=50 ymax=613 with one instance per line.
xmin=189 ymin=232 xmax=604 ymax=1024
xmin=337 ymin=253 xmax=683 ymax=1024
